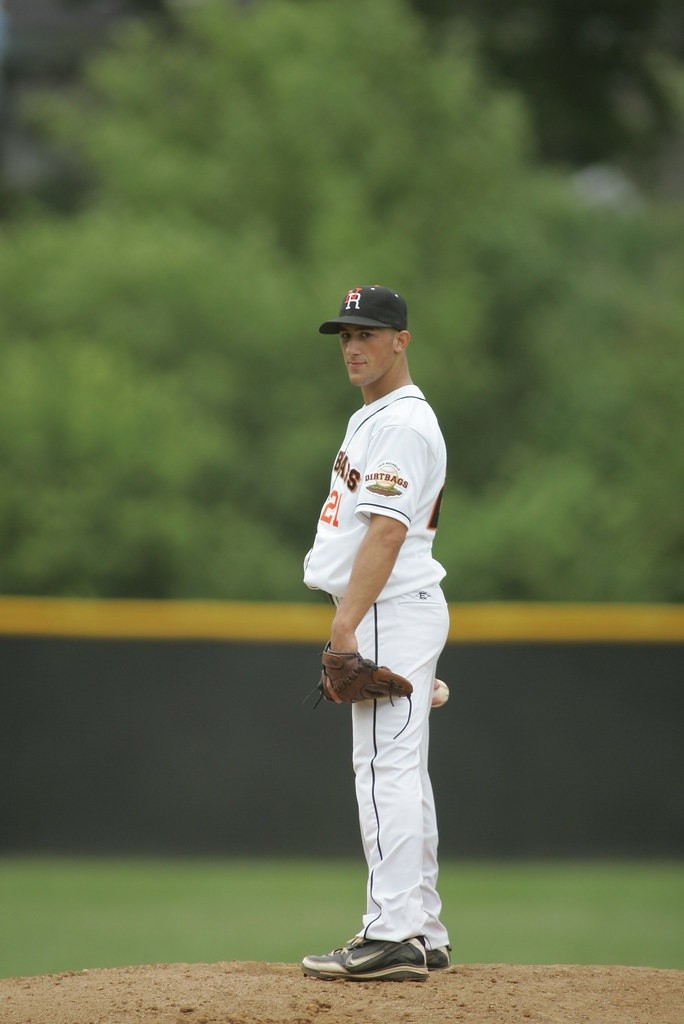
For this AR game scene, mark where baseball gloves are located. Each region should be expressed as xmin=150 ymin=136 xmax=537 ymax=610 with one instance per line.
xmin=304 ymin=641 xmax=414 ymax=703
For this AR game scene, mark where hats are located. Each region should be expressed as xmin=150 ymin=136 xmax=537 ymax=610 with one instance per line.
xmin=319 ymin=285 xmax=408 ymax=334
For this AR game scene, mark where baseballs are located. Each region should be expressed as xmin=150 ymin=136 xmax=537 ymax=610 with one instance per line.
xmin=431 ymin=679 xmax=450 ymax=707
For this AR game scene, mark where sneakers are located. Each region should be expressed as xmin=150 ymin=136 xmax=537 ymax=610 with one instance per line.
xmin=302 ymin=936 xmax=430 ymax=981
xmin=418 ymin=936 xmax=450 ymax=969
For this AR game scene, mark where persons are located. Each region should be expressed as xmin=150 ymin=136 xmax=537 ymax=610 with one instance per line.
xmin=304 ymin=286 xmax=453 ymax=985
